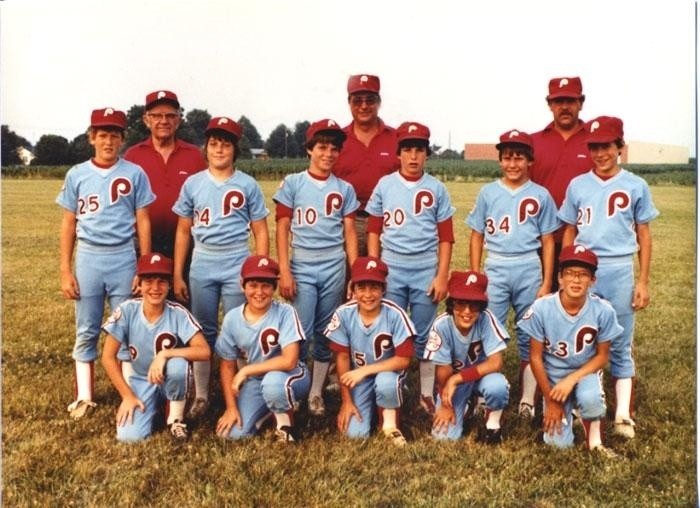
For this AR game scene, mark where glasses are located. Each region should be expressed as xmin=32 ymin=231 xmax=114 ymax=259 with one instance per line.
xmin=145 ymin=112 xmax=180 ymax=121
xmin=349 ymin=96 xmax=377 ymax=106
xmin=561 ymin=269 xmax=595 ymax=283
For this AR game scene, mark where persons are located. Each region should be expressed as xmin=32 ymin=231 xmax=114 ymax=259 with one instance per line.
xmin=123 ymin=90 xmax=208 ymax=348
xmin=555 ymin=116 xmax=661 ymax=437
xmin=365 ymin=121 xmax=457 ymax=414
xmin=322 ymin=256 xmax=418 ymax=448
xmin=463 ymin=130 xmax=567 ymax=423
xmin=100 ymin=253 xmax=213 ymax=444
xmin=527 ymin=76 xmax=594 ymax=291
xmin=330 ymin=74 xmax=401 ymax=254
xmin=214 ymin=255 xmax=312 ymax=443
xmin=54 ymin=107 xmax=157 ymax=420
xmin=516 ymin=245 xmax=625 ymax=459
xmin=272 ymin=119 xmax=361 ymax=417
xmin=172 ymin=116 xmax=270 ymax=420
xmin=423 ymin=271 xmax=512 ymax=443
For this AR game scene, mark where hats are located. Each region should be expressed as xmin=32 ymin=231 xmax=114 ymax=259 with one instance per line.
xmin=447 ymin=269 xmax=490 ymax=309
xmin=144 ymin=90 xmax=180 ymax=111
xmin=494 ymin=129 xmax=535 ymax=155
xmin=305 ymin=117 xmax=347 ymax=148
xmin=238 ymin=254 xmax=283 ymax=283
xmin=348 ymin=254 xmax=389 ymax=292
xmin=135 ymin=250 xmax=175 ymax=277
xmin=89 ymin=108 xmax=129 ymax=132
xmin=203 ymin=116 xmax=243 ymax=142
xmin=582 ymin=116 xmax=625 ymax=143
xmin=545 ymin=77 xmax=585 ymax=104
xmin=557 ymin=243 xmax=598 ymax=270
xmin=347 ymin=73 xmax=381 ymax=98
xmin=394 ymin=121 xmax=430 ymax=154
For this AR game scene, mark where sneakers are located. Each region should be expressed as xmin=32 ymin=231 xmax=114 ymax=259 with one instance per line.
xmin=381 ymin=427 xmax=408 ymax=451
xmin=419 ymin=393 xmax=437 ymax=415
xmin=588 ymin=443 xmax=626 ymax=466
xmin=481 ymin=424 xmax=504 ymax=444
xmin=517 ymin=401 xmax=535 ymax=424
xmin=167 ymin=420 xmax=193 ymax=452
xmin=183 ymin=396 xmax=209 ymax=423
xmin=305 ymin=395 xmax=327 ymax=418
xmin=65 ymin=397 xmax=99 ymax=421
xmin=611 ymin=415 xmax=636 ymax=440
xmin=271 ymin=424 xmax=299 ymax=448
